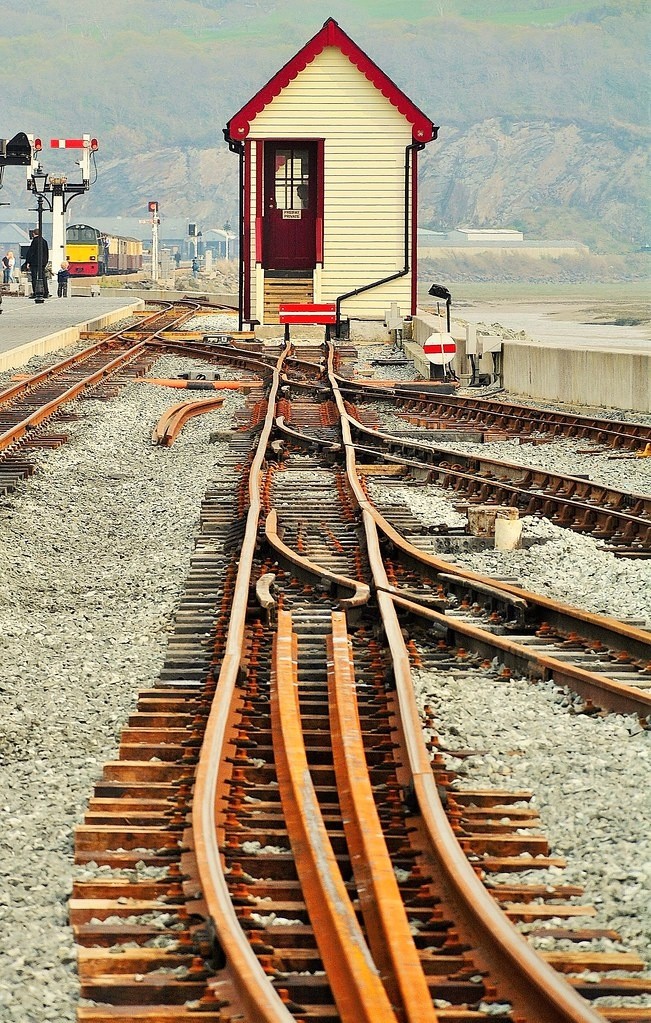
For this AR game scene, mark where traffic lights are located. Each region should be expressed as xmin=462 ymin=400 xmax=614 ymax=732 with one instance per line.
xmin=148 ymin=201 xmax=158 ymax=212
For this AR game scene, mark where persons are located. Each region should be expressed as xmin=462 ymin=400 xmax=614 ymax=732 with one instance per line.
xmin=174 ymin=251 xmax=181 ymax=267
xmin=57 ymin=260 xmax=71 ymax=298
xmin=191 ymin=259 xmax=199 ymax=280
xmin=26 ymin=228 xmax=52 ymax=298
xmin=1 ymin=251 xmax=16 ymax=283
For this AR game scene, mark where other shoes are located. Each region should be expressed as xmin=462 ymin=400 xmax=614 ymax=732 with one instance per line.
xmin=43 ymin=294 xmax=52 ymax=298
xmin=29 ymin=294 xmax=36 ymax=298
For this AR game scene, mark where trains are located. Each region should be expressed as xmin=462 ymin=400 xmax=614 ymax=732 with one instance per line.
xmin=66 ymin=223 xmax=144 ymax=277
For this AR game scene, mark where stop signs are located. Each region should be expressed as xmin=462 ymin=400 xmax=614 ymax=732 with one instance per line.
xmin=424 ymin=333 xmax=456 ymax=365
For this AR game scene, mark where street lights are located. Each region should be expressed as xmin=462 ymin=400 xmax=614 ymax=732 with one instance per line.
xmin=31 ymin=163 xmax=48 ymax=303
